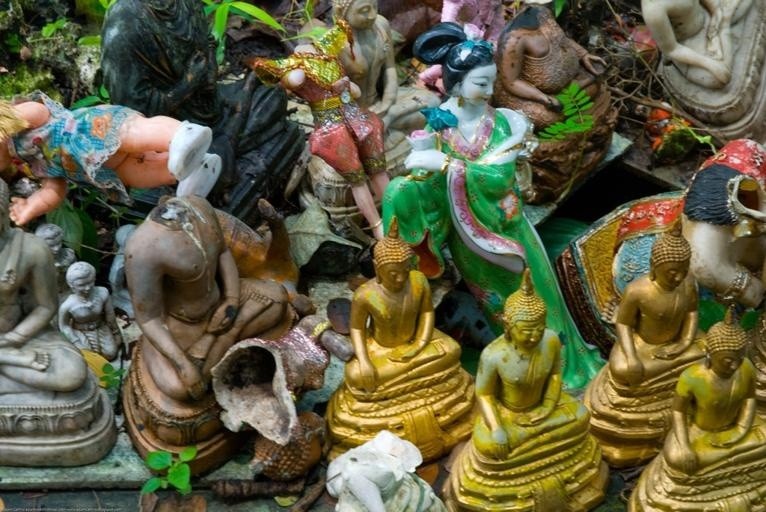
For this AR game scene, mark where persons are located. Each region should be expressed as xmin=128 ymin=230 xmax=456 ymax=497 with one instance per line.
xmin=1 ymin=1 xmax=766 ymax=512
